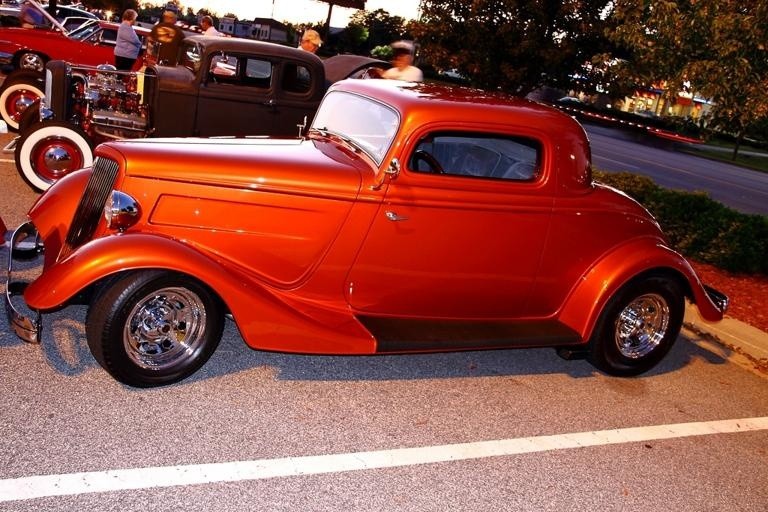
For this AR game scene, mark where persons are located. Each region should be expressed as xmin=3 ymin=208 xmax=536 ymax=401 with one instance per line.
xmin=296 ymin=29 xmax=325 ymax=79
xmin=114 ymin=8 xmax=143 ymax=80
xmin=698 ymin=110 xmax=706 ymax=128
xmin=378 ymin=42 xmax=423 ymax=81
xmin=201 ymin=15 xmax=220 ymax=72
xmin=146 ymin=6 xmax=186 ymax=58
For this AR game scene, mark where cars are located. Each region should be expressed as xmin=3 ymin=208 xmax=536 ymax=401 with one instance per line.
xmin=5 ymin=74 xmax=729 ymax=387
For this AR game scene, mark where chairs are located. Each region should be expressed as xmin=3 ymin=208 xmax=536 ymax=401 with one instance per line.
xmin=494 ymin=156 xmax=535 ymax=179
xmin=445 ymin=145 xmax=500 ymax=177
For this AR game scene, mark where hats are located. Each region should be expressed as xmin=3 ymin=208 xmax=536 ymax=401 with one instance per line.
xmin=392 ymin=41 xmax=414 ymax=55
xmin=303 ymin=29 xmax=323 ymax=46
